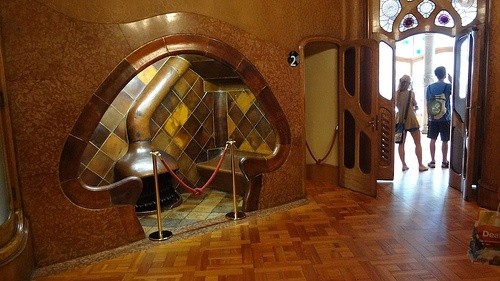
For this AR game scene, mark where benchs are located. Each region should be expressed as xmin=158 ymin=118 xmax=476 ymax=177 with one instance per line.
xmin=198 ymin=147 xmax=268 ymax=195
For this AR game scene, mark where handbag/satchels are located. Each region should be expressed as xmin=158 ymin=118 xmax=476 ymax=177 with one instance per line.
xmin=394 ymin=122 xmax=405 ymax=143
xmin=473 ymin=203 xmax=500 ymax=249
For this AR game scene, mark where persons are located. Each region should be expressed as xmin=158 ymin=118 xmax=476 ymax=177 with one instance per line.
xmin=425 ymin=66 xmax=452 ymax=168
xmin=395 ymin=75 xmax=429 ymax=172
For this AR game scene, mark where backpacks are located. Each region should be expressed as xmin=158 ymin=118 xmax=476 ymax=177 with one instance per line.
xmin=429 ymin=83 xmax=449 ymax=121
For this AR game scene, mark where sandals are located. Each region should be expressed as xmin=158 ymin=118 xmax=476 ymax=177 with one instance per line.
xmin=442 ymin=161 xmax=449 ymax=168
xmin=428 ymin=162 xmax=435 ymax=168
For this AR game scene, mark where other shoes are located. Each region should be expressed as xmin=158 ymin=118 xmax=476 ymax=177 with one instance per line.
xmin=419 ymin=165 xmax=428 ymax=171
xmin=402 ymin=165 xmax=409 ymax=171
